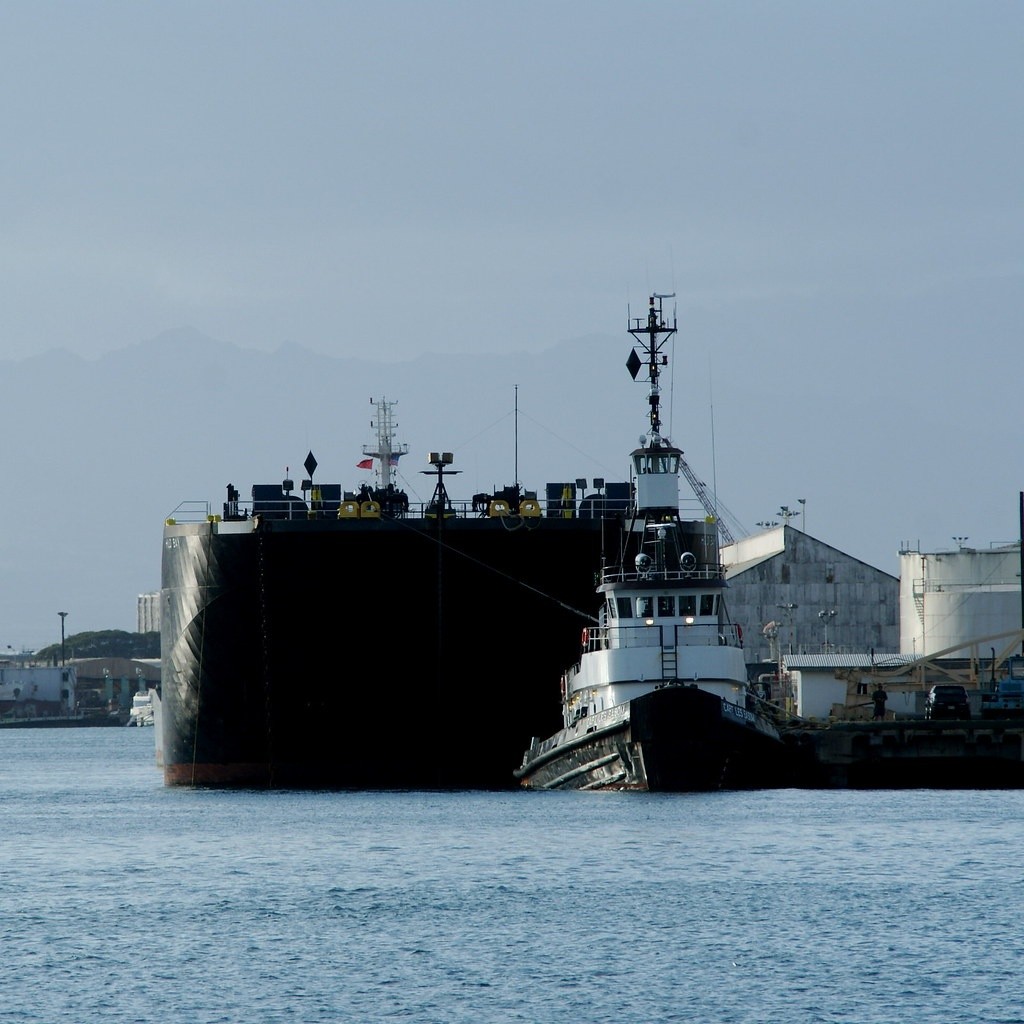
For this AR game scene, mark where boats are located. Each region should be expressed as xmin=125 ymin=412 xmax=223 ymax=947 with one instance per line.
xmin=129 ymin=691 xmax=154 ymax=727
xmin=158 ymin=479 xmax=722 ymax=792
xmin=510 ymin=293 xmax=785 ymax=795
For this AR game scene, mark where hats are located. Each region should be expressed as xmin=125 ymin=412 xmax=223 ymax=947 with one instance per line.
xmin=877 ymin=683 xmax=883 ymax=686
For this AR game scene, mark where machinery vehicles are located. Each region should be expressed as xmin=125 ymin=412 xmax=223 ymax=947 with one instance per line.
xmin=979 ymin=648 xmax=1024 ymax=719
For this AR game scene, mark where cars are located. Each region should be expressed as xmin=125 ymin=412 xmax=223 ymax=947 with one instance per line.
xmin=31 ymin=663 xmax=40 ymax=667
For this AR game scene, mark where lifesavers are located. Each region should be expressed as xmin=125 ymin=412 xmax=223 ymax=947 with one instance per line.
xmin=581 ymin=627 xmax=590 ymax=647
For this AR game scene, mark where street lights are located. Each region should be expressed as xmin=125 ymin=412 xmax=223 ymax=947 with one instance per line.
xmin=755 ymin=521 xmax=780 ymax=529
xmin=58 ymin=612 xmax=68 ymax=666
xmin=775 ymin=602 xmax=798 ymax=653
xmin=818 ymin=610 xmax=838 ymax=652
xmin=798 ymin=498 xmax=806 ymax=532
xmin=951 ymin=536 xmax=970 ymax=548
xmin=775 ymin=507 xmax=799 ymax=526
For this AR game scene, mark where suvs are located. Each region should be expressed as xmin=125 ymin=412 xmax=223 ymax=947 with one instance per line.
xmin=924 ymin=684 xmax=972 ymax=720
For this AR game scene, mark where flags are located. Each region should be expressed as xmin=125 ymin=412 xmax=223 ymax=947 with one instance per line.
xmin=391 ymin=454 xmax=399 ymax=465
xmin=356 ymin=460 xmax=372 ymax=469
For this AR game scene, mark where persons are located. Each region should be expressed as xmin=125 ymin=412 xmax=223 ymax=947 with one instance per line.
xmin=872 ymin=683 xmax=888 ymax=721
